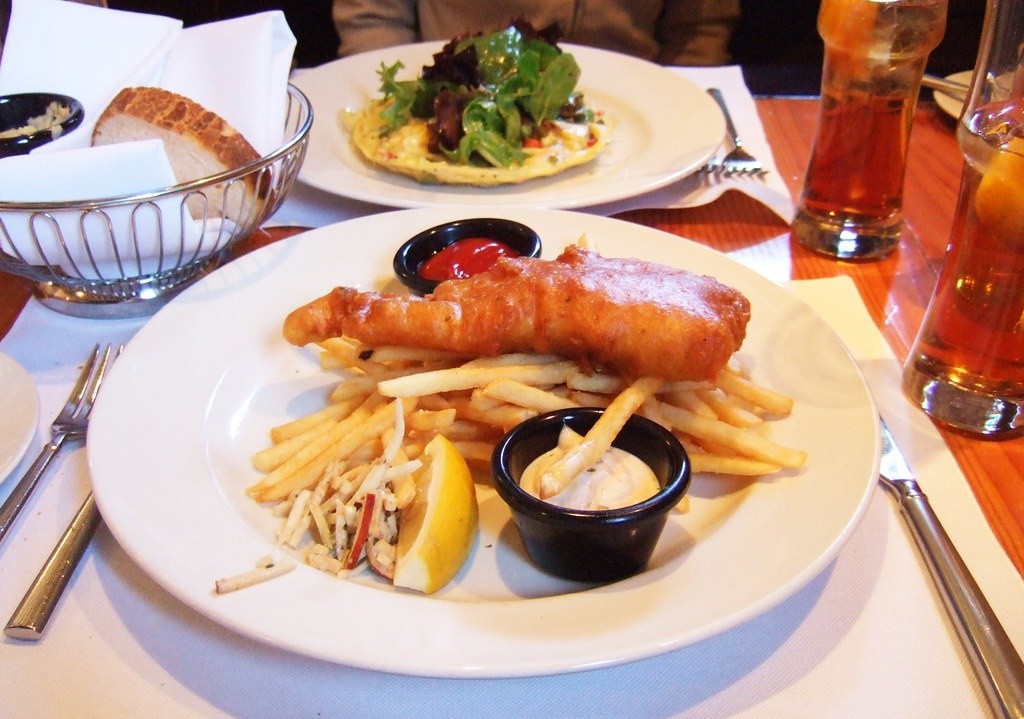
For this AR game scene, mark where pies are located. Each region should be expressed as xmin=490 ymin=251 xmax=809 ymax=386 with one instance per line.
xmin=340 ymin=98 xmax=607 ymax=188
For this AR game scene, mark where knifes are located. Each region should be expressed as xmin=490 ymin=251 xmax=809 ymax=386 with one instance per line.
xmin=878 ymin=414 xmax=1024 ymax=719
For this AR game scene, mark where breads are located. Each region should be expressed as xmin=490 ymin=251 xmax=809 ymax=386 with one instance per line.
xmin=91 ymin=87 xmax=275 ymax=227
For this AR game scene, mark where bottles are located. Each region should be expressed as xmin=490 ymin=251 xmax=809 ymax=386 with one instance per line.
xmin=897 ymin=1 xmax=1024 ymax=434
xmin=787 ymin=0 xmax=948 ymax=260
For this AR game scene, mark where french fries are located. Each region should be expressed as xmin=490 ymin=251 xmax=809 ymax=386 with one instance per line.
xmin=213 ymin=336 xmax=808 ymax=595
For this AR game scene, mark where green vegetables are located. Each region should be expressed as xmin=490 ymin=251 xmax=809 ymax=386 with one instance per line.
xmin=376 ymin=20 xmax=581 ymax=167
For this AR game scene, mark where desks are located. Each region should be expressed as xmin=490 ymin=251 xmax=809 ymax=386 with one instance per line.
xmin=0 ymin=98 xmax=1024 ymax=717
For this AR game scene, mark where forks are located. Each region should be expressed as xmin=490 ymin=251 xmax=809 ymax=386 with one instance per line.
xmin=695 ymin=156 xmax=718 ymax=173
xmin=0 ymin=342 xmax=124 ymax=543
xmin=706 ymin=88 xmax=770 ymax=178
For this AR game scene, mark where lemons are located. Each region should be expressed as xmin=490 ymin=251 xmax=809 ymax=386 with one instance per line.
xmin=393 ymin=433 xmax=478 ymax=594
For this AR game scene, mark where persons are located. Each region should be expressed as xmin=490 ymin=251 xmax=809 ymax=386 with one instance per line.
xmin=333 ymin=0 xmax=739 ymax=68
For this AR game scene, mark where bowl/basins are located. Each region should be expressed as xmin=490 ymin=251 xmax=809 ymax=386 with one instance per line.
xmin=392 ymin=217 xmax=542 ymax=300
xmin=490 ymin=407 xmax=692 ymax=584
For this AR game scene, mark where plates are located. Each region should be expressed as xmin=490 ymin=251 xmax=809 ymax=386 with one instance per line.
xmin=0 ymin=353 xmax=41 ymax=484
xmin=933 ymin=69 xmax=1016 ymax=122
xmin=281 ymin=40 xmax=728 ymax=211
xmin=85 ymin=213 xmax=883 ymax=681
